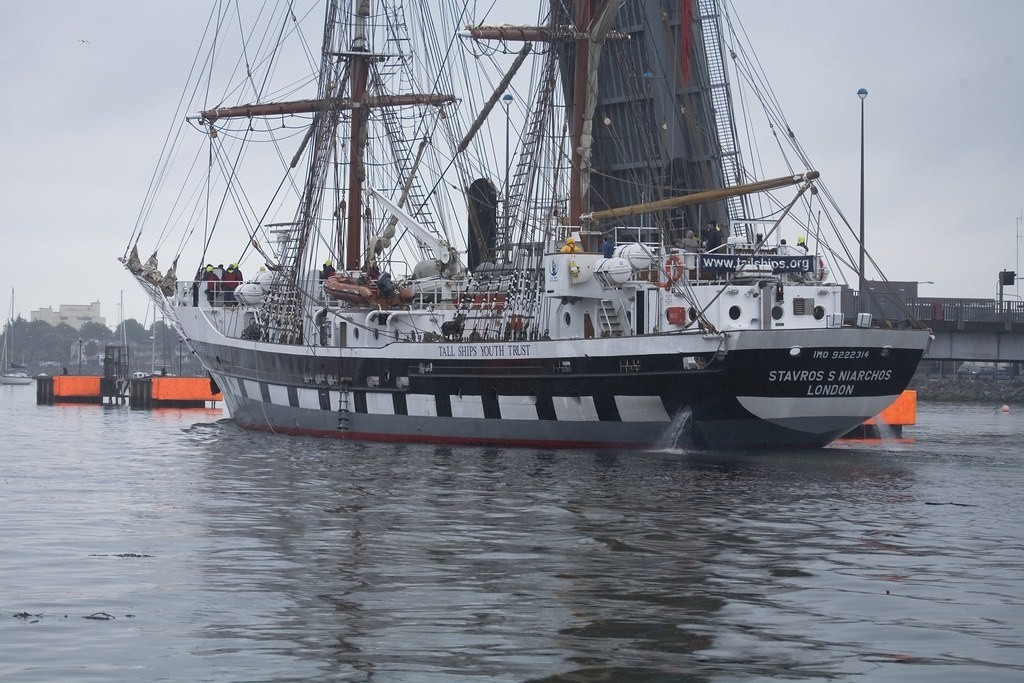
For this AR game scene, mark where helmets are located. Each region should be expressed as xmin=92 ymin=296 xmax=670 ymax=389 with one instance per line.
xmin=716 ymin=225 xmax=720 ymax=231
xmin=799 ymin=237 xmax=804 ymax=243
xmin=260 ymin=267 xmax=265 ymax=271
xmin=234 ymin=263 xmax=238 ymax=268
xmin=326 ymin=261 xmax=331 ymax=266
xmin=207 ymin=266 xmax=212 ymax=271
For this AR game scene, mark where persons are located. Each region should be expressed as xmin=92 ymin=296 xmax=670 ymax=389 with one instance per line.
xmin=676 ymin=221 xmax=723 ymax=279
xmin=320 ymin=260 xmax=380 ymax=280
xmin=600 ymin=237 xmax=614 ymax=258
xmin=777 ymin=235 xmax=809 ymax=256
xmin=193 ymin=263 xmax=265 ymax=307
xmin=561 ymin=237 xmax=582 ymax=253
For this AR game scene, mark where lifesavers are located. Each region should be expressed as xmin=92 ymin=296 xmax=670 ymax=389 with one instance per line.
xmin=664 ymin=254 xmax=685 ymax=282
xmin=817 ymin=258 xmax=827 ymax=282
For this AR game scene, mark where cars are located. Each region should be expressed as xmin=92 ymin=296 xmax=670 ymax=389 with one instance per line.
xmin=132 ymin=371 xmax=176 ymax=379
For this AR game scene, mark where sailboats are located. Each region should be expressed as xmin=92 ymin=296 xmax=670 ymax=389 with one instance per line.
xmin=116 ymin=0 xmax=932 ymax=453
xmin=0 ymin=285 xmax=35 ymax=385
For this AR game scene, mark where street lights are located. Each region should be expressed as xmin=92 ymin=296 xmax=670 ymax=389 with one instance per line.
xmin=79 ymin=340 xmax=82 ymax=376
xmin=857 ymin=87 xmax=869 ymax=291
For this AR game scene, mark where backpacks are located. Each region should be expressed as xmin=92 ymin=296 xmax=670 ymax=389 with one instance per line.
xmin=567 ymin=244 xmax=575 ymax=252
